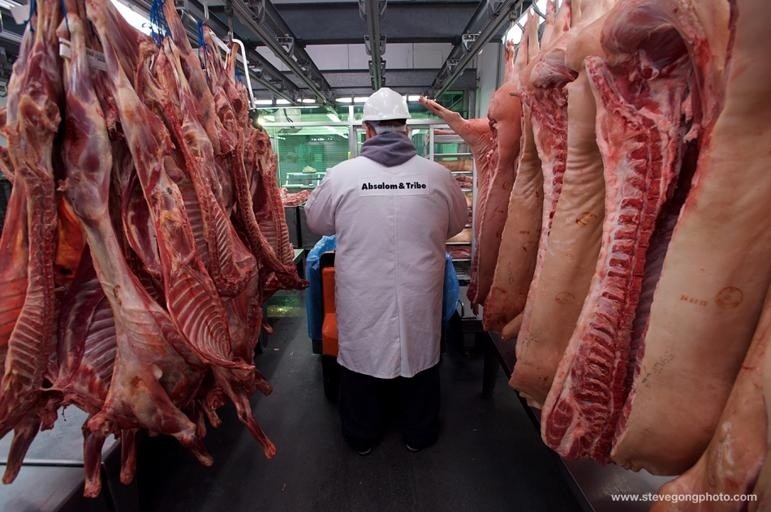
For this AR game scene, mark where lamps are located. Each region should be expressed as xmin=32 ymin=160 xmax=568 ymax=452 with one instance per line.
xmin=502 ymin=0 xmax=562 ymax=46
xmin=111 ymin=0 xmax=167 ymax=41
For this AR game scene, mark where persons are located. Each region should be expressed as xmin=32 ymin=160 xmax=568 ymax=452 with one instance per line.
xmin=303 ymin=88 xmax=468 ymax=457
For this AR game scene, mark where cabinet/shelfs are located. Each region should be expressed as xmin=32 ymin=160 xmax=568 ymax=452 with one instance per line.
xmin=424 ymin=123 xmax=477 ymax=282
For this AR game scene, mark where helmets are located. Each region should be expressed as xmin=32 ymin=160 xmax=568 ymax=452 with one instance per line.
xmin=362 ymin=86 xmax=411 ymax=122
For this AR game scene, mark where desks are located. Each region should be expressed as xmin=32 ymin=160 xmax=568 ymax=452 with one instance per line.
xmin=293 ymin=248 xmax=305 ymax=280
